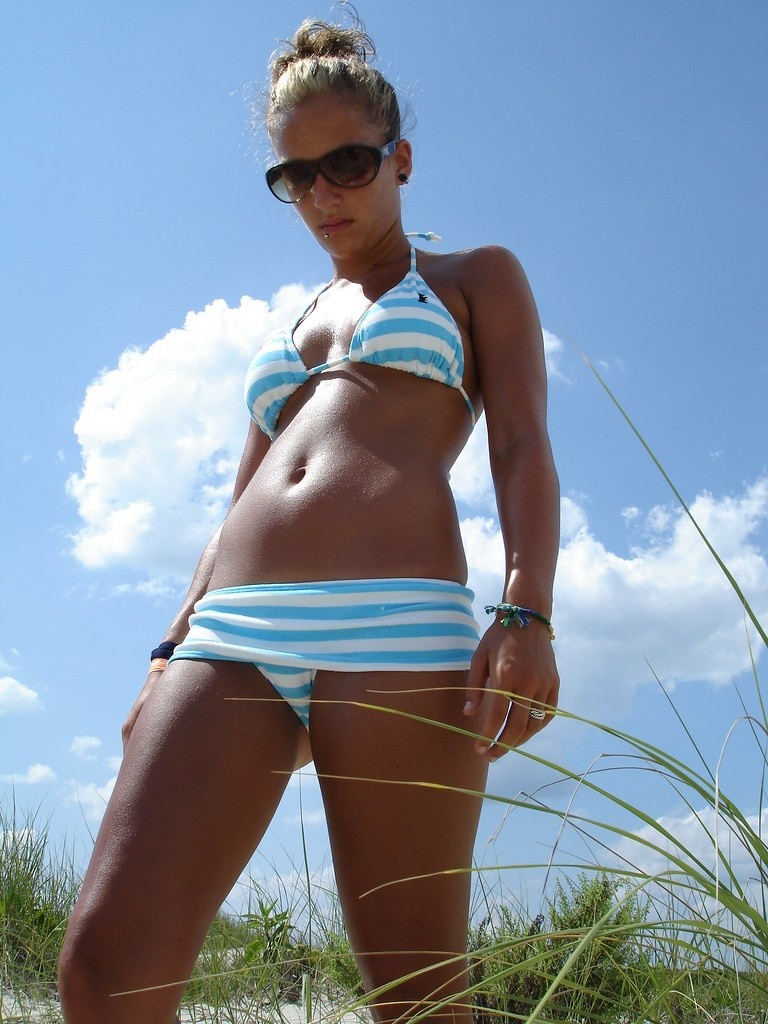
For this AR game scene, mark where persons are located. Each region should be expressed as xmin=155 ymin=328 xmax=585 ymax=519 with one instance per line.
xmin=56 ymin=20 xmax=560 ymax=1024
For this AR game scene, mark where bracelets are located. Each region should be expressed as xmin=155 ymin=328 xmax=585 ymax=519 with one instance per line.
xmin=148 ymin=658 xmax=168 ymax=674
xmin=151 ymin=641 xmax=179 ymax=659
xmin=484 ymin=603 xmax=556 ymax=640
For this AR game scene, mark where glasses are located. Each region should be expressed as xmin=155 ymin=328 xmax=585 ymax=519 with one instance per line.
xmin=265 ymin=139 xmax=400 ymax=204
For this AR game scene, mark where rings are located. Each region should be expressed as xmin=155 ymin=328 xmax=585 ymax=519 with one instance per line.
xmin=530 ymin=707 xmax=545 ymax=719
